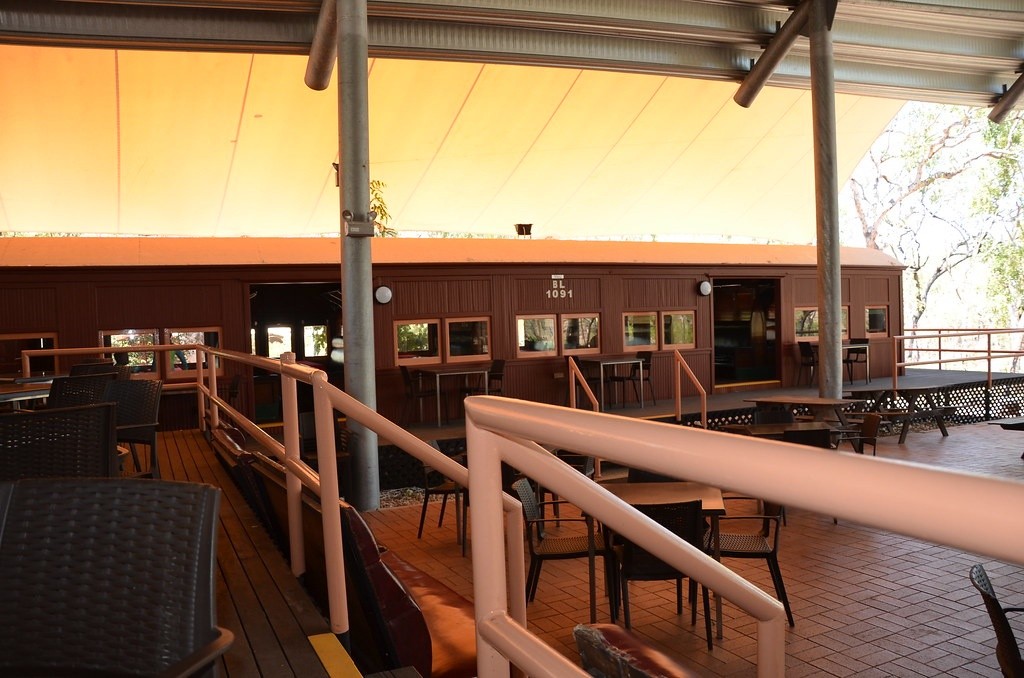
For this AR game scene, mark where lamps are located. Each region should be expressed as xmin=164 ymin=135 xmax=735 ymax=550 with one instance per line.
xmin=513 ymin=222 xmax=535 ymax=240
xmin=331 ymin=162 xmax=339 ymax=187
xmin=341 ymin=209 xmax=378 ymax=237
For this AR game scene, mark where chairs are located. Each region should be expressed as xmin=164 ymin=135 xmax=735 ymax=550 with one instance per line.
xmin=540 ymin=454 xmax=601 ymax=532
xmin=101 ymin=379 xmax=163 ymax=480
xmin=845 ymin=338 xmax=871 ymax=384
xmin=0 ymin=401 xmax=121 ymax=479
xmin=398 ymin=364 xmax=448 ymax=426
xmin=46 ymin=373 xmax=118 ymax=408
xmin=416 ymin=440 xmax=464 ymax=545
xmin=564 ymin=355 xmax=611 ymax=411
xmin=781 ymin=429 xmax=839 ymax=527
xmin=512 ymin=478 xmax=607 ymax=607
xmin=69 ymin=361 xmax=114 ymax=376
xmin=720 ymin=424 xmax=753 ymax=436
xmin=796 ymin=341 xmax=820 ymax=388
xmin=0 ymin=479 xmax=236 ymax=678
xmin=703 ymin=495 xmax=797 ymax=631
xmin=613 ymin=350 xmax=657 ymax=408
xmin=628 ymin=467 xmax=685 ymax=483
xmin=837 ymin=415 xmax=882 ymax=456
xmin=969 ymin=563 xmax=1024 ymax=678
xmin=92 ymin=366 xmax=132 ymax=380
xmin=613 ymin=499 xmax=714 ymax=653
xmin=459 ymin=360 xmax=506 ymax=417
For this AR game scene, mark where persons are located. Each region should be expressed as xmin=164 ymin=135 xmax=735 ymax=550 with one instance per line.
xmin=114 ymin=352 xmax=134 ymax=372
xmin=152 ymin=333 xmax=188 ymax=372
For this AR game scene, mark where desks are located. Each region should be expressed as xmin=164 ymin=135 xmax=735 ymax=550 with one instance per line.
xmin=0 ymin=384 xmax=54 ymax=413
xmin=577 ymin=357 xmax=645 ymax=412
xmin=461 ymin=446 xmax=556 ymax=555
xmin=745 ymin=396 xmax=861 ymax=454
xmin=415 ymin=367 xmax=492 ymax=428
xmin=14 ymin=374 xmax=69 ymax=385
xmin=583 ymin=482 xmax=727 ymax=641
xmin=812 ymin=344 xmax=870 ymax=385
xmin=844 ymin=384 xmax=956 ymax=444
xmin=747 ymin=421 xmax=841 ymax=437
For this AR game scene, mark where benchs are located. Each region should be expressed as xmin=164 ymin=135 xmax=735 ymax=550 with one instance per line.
xmin=845 ymin=411 xmax=909 ymax=425
xmin=794 ymin=415 xmax=892 ymax=426
xmin=889 ymin=405 xmax=966 ymax=416
